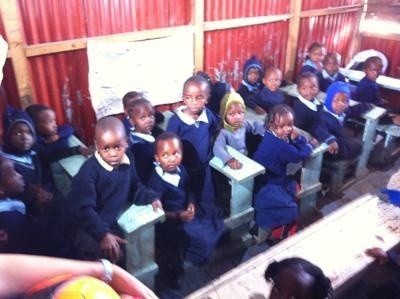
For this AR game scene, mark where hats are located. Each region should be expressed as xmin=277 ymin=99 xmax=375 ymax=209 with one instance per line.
xmin=324 ymin=81 xmax=351 ymax=114
xmin=5 ymin=107 xmax=36 ymax=146
xmin=219 ymin=87 xmax=245 ymax=131
xmin=244 ymin=55 xmax=262 ymax=81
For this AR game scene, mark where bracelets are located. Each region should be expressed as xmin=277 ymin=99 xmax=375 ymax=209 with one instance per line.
xmin=100 ymin=257 xmax=114 ymax=283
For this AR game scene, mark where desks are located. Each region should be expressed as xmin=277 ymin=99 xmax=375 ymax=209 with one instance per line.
xmin=0 ymin=65 xmax=400 ymax=292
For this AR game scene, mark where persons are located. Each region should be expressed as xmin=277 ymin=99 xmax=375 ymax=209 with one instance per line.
xmin=265 ymin=258 xmax=333 ymax=299
xmin=121 ymin=43 xmax=398 ymax=271
xmin=1 ymin=158 xmax=52 ymax=245
xmin=1 ymin=254 xmax=158 ymax=299
xmin=61 ymin=115 xmax=161 ymax=261
xmin=0 ymin=106 xmax=53 ymax=189
xmin=26 ymin=105 xmax=93 ymax=158
